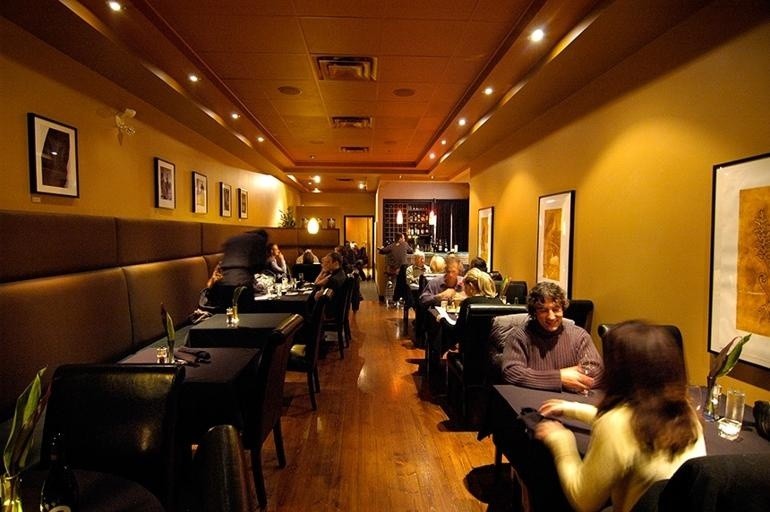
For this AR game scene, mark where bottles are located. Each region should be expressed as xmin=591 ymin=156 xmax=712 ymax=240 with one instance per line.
xmin=225 ymin=307 xmax=233 ymax=326
xmin=156 ymin=346 xmax=166 ymax=362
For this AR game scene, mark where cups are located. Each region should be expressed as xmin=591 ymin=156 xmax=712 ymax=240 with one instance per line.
xmin=714 ymin=416 xmax=742 ymax=442
xmin=724 ymin=389 xmax=745 ymax=422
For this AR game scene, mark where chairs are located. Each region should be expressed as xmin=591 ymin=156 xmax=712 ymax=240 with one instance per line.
xmin=627 ymin=453 xmax=766 ymax=508
xmin=443 ymin=299 xmax=594 ymax=430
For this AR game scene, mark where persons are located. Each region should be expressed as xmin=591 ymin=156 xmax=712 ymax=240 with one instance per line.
xmin=212 ymin=230 xmax=278 ymax=314
xmin=296 ymin=249 xmax=319 ymax=264
xmin=351 ymin=243 xmax=369 ymax=281
xmin=344 ymin=240 xmax=356 ymax=262
xmin=447 ymin=252 xmax=469 ymax=274
xmin=501 ymin=282 xmax=605 ymax=396
xmin=379 ymin=233 xmax=414 ymax=307
xmin=535 ymin=320 xmax=708 ymax=510
xmin=418 ymin=258 xmax=467 ymax=309
xmin=429 ymin=255 xmax=447 ymax=273
xmin=297 ymin=252 xmax=348 ymax=345
xmin=335 ymin=246 xmax=351 ymax=273
xmin=443 ymin=267 xmax=504 ymax=360
xmin=405 ymin=250 xmax=431 ymax=286
xmin=265 ymin=244 xmax=287 ymax=283
xmin=471 ymin=258 xmax=502 ymax=280
xmin=314 ymin=256 xmax=331 ymax=286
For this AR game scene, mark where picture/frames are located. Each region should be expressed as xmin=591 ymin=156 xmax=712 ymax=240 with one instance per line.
xmin=220 ymin=181 xmax=232 ymax=218
xmin=26 ymin=111 xmax=80 ymax=199
xmin=155 ymin=156 xmax=177 ymax=210
xmin=476 ymin=206 xmax=493 ymax=274
xmin=191 ymin=171 xmax=208 ymax=215
xmin=237 ymin=186 xmax=248 ymax=219
xmin=707 ymin=151 xmax=769 ymax=372
xmin=536 ymin=189 xmax=576 ymax=299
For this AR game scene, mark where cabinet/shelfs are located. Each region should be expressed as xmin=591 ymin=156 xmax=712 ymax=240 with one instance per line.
xmin=383 ymin=198 xmax=468 ymax=252
